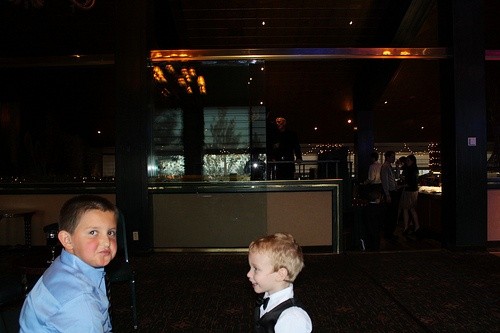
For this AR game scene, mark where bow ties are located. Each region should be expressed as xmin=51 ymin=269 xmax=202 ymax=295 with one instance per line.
xmin=255 ymin=297 xmax=270 ymax=310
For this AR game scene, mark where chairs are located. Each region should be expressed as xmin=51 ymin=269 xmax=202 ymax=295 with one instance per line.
xmin=25 ymin=210 xmax=138 ymax=330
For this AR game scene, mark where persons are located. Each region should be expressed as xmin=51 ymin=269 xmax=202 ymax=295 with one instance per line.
xmin=363 ymin=151 xmax=382 ymax=191
xmin=18 ymin=195 xmax=119 ymax=333
xmin=246 ymin=232 xmax=313 ymax=333
xmin=362 ymin=188 xmax=386 ymax=252
xmin=380 ymin=150 xmax=401 ymax=242
xmin=155 ymin=159 xmax=175 ymax=178
xmin=266 ymin=117 xmax=302 ymax=180
xmin=394 ymin=156 xmax=410 ymax=228
xmin=244 ymin=153 xmax=266 ymax=180
xmin=400 ymin=155 xmax=421 ymax=236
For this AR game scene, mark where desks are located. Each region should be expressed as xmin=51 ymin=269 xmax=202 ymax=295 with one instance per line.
xmin=0 ymin=209 xmax=37 ymax=249
